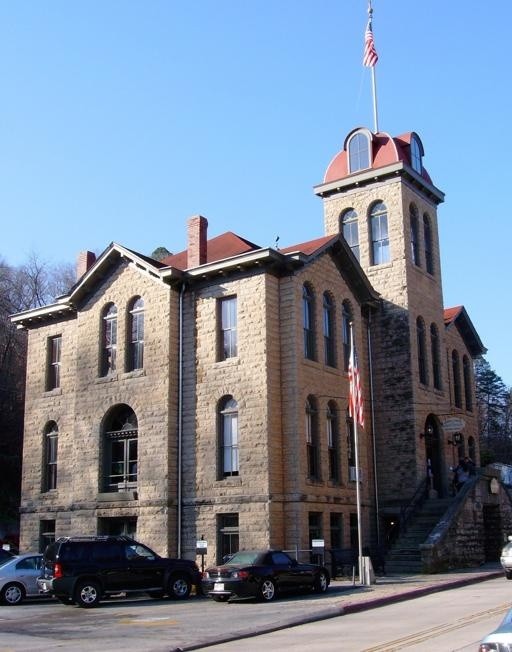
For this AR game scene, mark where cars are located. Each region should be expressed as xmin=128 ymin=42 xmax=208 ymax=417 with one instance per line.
xmin=500 ymin=535 xmax=512 ymax=580
xmin=478 ymin=607 xmax=512 ymax=652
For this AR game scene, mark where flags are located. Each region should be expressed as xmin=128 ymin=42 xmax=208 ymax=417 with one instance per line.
xmin=348 ymin=341 xmax=364 ymax=433
xmin=363 ymin=21 xmax=378 ymax=69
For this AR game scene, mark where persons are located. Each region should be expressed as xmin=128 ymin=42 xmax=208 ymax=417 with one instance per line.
xmin=448 ymin=457 xmax=475 ymax=496
